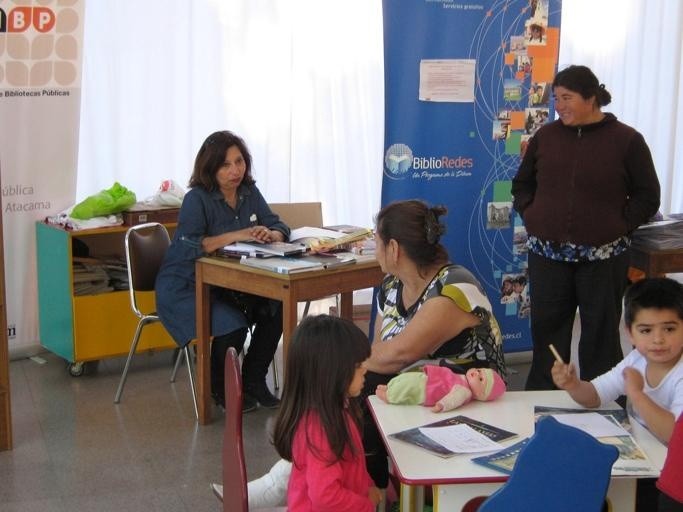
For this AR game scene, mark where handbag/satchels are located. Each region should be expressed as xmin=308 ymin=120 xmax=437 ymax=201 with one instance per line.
xmin=69 ymin=183 xmax=136 ymax=220
xmin=45 ymin=198 xmax=124 ymax=232
xmin=142 ymin=179 xmax=187 ymax=209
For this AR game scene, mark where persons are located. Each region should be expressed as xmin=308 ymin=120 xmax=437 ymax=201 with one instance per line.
xmin=508 ymin=63 xmax=661 ymax=409
xmin=208 ymin=198 xmax=506 ymax=512
xmin=153 ymin=128 xmax=290 ymax=413
xmin=374 ymin=364 xmax=506 ymax=413
xmin=512 ymin=276 xmax=527 ymax=303
xmin=268 ymin=311 xmax=382 ymax=512
xmin=550 ymin=275 xmax=682 ymax=511
xmin=522 ymin=20 xmax=549 ymax=136
xmin=501 ymin=276 xmax=513 ymax=303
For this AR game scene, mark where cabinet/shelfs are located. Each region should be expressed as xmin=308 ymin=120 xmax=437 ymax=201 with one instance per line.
xmin=34 ymin=221 xmax=192 ymax=363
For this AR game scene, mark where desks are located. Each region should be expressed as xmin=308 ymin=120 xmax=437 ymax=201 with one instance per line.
xmin=194 ymin=256 xmax=389 ymax=423
xmin=366 ymin=389 xmax=660 ymax=511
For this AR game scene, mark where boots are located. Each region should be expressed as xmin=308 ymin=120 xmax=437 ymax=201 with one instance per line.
xmin=212 ymin=457 xmax=292 ymax=509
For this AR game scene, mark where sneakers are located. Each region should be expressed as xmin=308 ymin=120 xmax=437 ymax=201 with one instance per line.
xmin=242 ymin=378 xmax=281 ymax=407
xmin=212 ymin=391 xmax=258 ymax=413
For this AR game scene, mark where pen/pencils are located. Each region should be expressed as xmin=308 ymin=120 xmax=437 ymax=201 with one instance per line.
xmin=549 ymin=343 xmax=564 ymax=364
xmin=318 ymin=252 xmax=338 ymax=257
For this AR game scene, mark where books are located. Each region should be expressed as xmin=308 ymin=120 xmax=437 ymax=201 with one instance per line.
xmin=71 ymin=251 xmax=129 ymax=296
xmin=532 ymin=414 xmax=656 ymax=477
xmin=470 ymin=435 xmax=531 ymax=476
xmin=534 ymin=404 xmax=634 ymax=435
xmin=387 ymin=414 xmax=519 ymax=459
xmin=215 ymin=224 xmax=376 ymax=275
